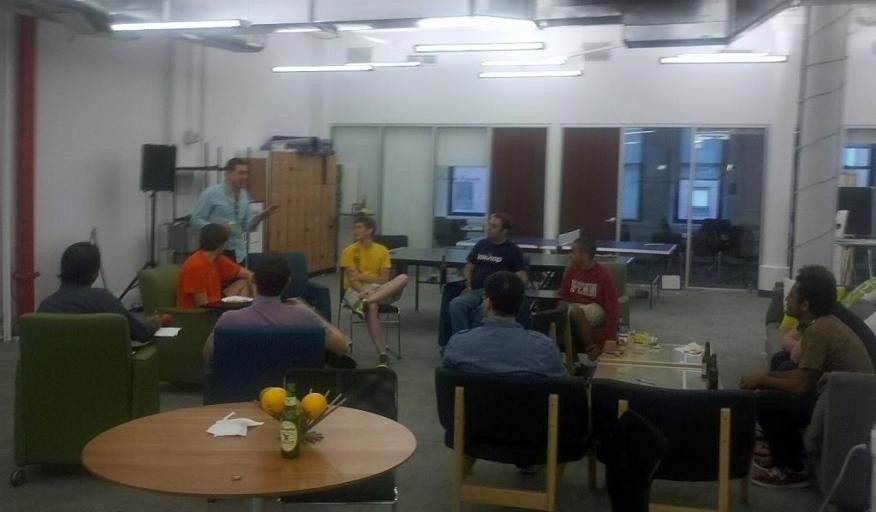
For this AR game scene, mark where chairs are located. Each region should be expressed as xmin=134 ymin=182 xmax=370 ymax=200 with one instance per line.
xmin=243 ymin=250 xmax=332 ymax=325
xmin=806 ymin=371 xmax=875 ymax=511
xmin=586 ymin=374 xmax=757 ymax=512
xmin=337 ymin=269 xmax=404 ymax=361
xmin=374 ymin=233 xmax=408 ymax=273
xmin=530 ymin=305 xmax=573 ymax=365
xmin=201 ymin=324 xmax=325 ymax=404
xmin=436 ymin=279 xmax=466 ymax=355
xmin=138 ymin=263 xmax=225 ymax=391
xmin=275 ymin=366 xmax=397 ymax=510
xmin=433 ymin=363 xmax=590 ymax=512
xmin=16 ymin=311 xmax=162 ymax=485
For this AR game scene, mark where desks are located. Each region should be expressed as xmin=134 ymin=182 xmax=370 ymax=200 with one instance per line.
xmin=389 ymin=246 xmax=636 ymax=313
xmin=200 ymin=296 xmax=253 ymax=312
xmin=79 ymin=400 xmax=417 ymax=510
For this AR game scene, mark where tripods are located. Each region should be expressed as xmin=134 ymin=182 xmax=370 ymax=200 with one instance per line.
xmin=117 ymin=191 xmax=157 ymax=302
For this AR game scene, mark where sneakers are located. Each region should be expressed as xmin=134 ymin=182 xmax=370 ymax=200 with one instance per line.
xmin=751 ymin=455 xmax=777 ymax=472
xmin=748 ymin=464 xmax=811 ymax=490
xmin=350 ymin=297 xmax=369 ymax=321
xmin=376 ymin=352 xmax=390 ymax=368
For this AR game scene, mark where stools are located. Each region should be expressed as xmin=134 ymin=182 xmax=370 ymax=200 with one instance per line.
xmin=837 ymin=238 xmax=874 ymax=286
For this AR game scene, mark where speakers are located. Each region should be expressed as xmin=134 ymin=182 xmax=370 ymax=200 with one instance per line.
xmin=144 ymin=144 xmax=175 ymax=191
xmin=838 ymin=186 xmax=876 ymax=239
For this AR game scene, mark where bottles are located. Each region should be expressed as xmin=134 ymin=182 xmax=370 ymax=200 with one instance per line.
xmin=701 ymin=340 xmax=712 ymax=378
xmin=280 ymin=383 xmax=299 ymax=456
xmin=707 ymin=352 xmax=719 ymax=388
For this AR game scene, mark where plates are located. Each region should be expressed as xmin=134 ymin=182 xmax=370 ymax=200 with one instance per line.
xmin=220 ymin=295 xmax=253 ymax=303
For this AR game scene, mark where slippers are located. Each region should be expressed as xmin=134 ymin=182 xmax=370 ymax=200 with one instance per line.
xmin=752 ymin=427 xmax=765 ymax=440
xmin=751 ymin=442 xmax=771 ymax=456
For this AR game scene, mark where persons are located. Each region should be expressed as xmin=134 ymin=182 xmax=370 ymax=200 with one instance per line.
xmin=554 ymin=235 xmax=620 ymax=373
xmin=738 ymin=265 xmax=876 ymax=490
xmin=202 ymin=250 xmax=348 ymax=359
xmin=340 ymin=216 xmax=408 ymax=368
xmin=448 ymin=212 xmax=529 ymax=333
xmin=190 ymin=157 xmax=279 ymax=270
xmin=36 ymin=240 xmax=162 ymax=355
xmin=442 ymin=271 xmax=571 ymax=384
xmin=176 ymin=222 xmax=258 ymax=310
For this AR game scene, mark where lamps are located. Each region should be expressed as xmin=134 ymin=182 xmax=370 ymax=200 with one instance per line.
xmin=109 ymin=7 xmax=800 ymax=83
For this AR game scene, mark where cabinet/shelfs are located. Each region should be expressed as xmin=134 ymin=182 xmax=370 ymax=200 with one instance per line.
xmin=241 ymin=148 xmax=336 ymax=276
